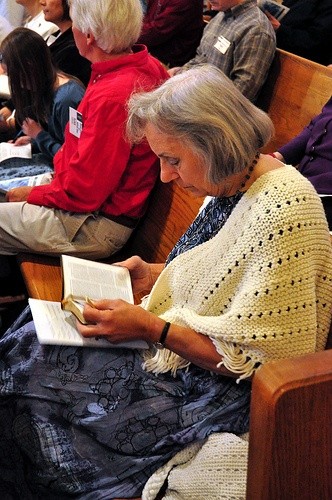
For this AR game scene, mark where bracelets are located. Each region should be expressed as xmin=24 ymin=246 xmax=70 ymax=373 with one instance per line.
xmin=155 ymin=322 xmax=171 ymax=349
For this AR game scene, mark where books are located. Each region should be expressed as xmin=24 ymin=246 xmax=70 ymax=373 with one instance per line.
xmin=59 ymin=253 xmax=134 ymax=328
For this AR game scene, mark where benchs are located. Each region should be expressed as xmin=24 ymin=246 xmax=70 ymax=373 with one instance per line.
xmin=17 ymin=47 xmax=332 ymax=499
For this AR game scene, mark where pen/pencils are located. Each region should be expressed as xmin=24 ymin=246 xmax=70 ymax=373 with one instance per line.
xmin=12 ymin=129 xmax=22 ymax=143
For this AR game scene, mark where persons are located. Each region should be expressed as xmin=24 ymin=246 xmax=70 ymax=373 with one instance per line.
xmin=135 ymin=1 xmax=203 ymax=68
xmin=0 ymin=0 xmax=92 ymax=148
xmin=0 ymin=28 xmax=87 ymax=188
xmin=0 ymin=0 xmax=61 ymax=49
xmin=167 ymin=0 xmax=281 ymax=103
xmin=0 ymin=63 xmax=332 ymax=500
xmin=0 ymin=1 xmax=174 ymax=293
